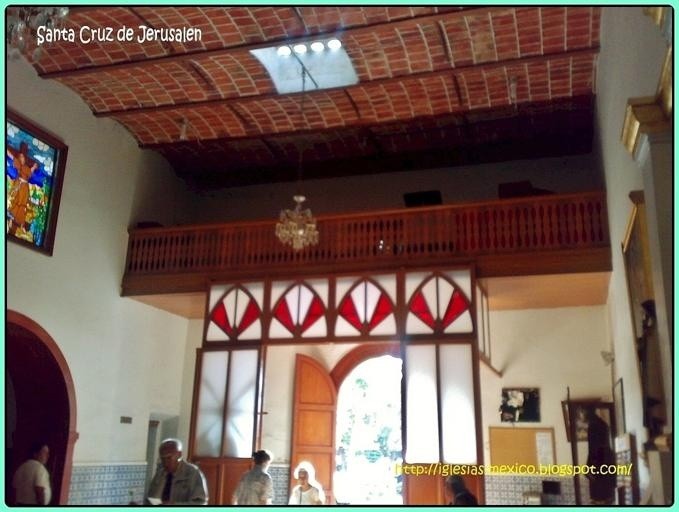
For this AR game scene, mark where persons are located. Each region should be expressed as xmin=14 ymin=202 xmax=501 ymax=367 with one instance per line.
xmin=288 ymin=461 xmax=327 ymax=507
xmin=12 ymin=439 xmax=54 ymax=506
xmin=231 ymin=449 xmax=276 ymax=505
xmin=578 ymin=405 xmax=617 ymax=505
xmin=7 ymin=140 xmax=41 ymax=238
xmin=442 ymin=472 xmax=477 ymax=505
xmin=143 ymin=437 xmax=210 ymax=506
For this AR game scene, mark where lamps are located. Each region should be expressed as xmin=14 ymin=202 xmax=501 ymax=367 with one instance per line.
xmin=275 ymin=67 xmax=319 ymax=250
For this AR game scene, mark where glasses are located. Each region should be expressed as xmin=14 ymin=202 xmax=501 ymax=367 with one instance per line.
xmin=158 ymin=451 xmax=178 ymax=463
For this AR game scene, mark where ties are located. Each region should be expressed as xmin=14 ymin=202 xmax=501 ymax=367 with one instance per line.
xmin=161 ymin=473 xmax=174 ymax=503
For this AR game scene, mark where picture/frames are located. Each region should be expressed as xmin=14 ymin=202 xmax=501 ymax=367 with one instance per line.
xmin=6 ymin=106 xmax=68 ymax=256
xmin=562 ymin=398 xmax=603 ymax=440
